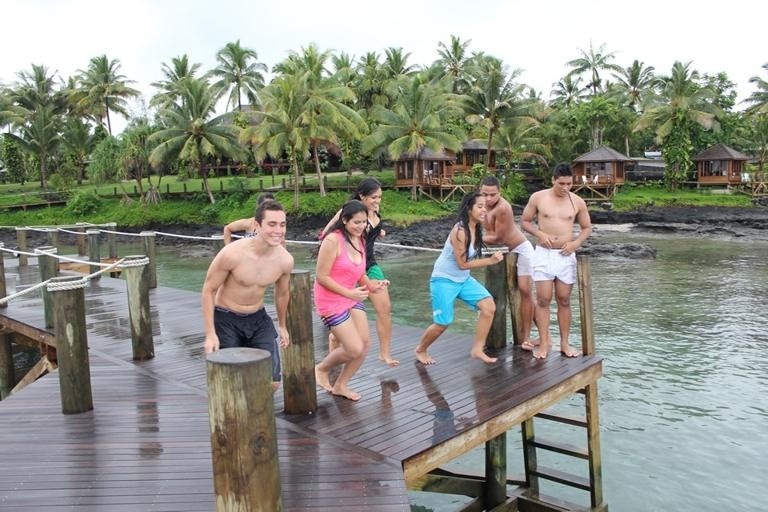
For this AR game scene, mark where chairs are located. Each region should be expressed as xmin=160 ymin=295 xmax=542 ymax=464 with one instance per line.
xmin=582 ymin=175 xmax=588 ymax=184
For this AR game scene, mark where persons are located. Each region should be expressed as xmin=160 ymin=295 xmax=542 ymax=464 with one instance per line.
xmin=310 ymin=199 xmax=391 ymax=402
xmin=414 ymin=191 xmax=505 ymax=366
xmin=318 ymin=177 xmax=401 ymax=368
xmin=202 ymin=200 xmax=295 ymax=394
xmin=520 ymin=161 xmax=592 ymax=359
xmin=225 ymin=192 xmax=286 ymax=249
xmin=479 ymin=175 xmax=552 ymax=351
